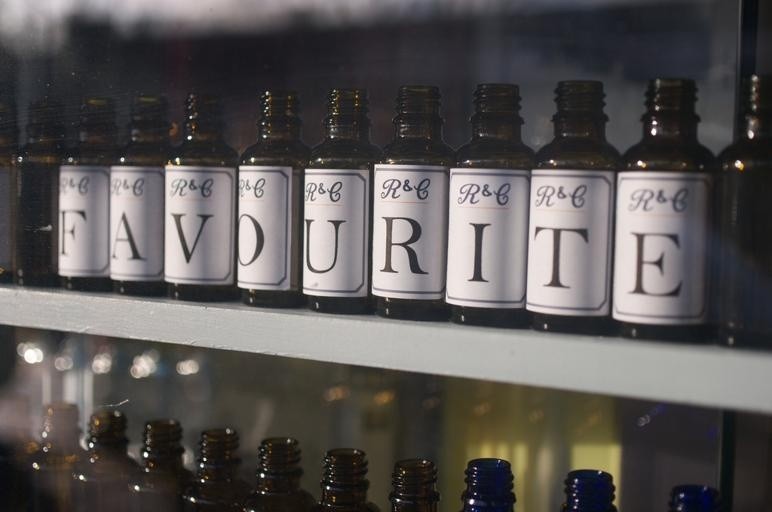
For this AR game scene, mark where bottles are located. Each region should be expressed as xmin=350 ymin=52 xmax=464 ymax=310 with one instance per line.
xmin=611 ymin=76 xmax=718 ymax=342
xmin=525 ymin=78 xmax=622 ymax=342
xmin=372 ymin=83 xmax=447 ymax=321
xmin=444 ymin=82 xmax=528 ymax=329
xmin=17 ymin=76 xmax=58 ymax=287
xmin=57 ymin=80 xmax=109 ymax=289
xmin=0 ymin=94 xmax=16 ymax=284
xmin=304 ymin=85 xmax=379 ymax=316
xmin=237 ymin=85 xmax=309 ymax=309
xmin=165 ymin=80 xmax=236 ymax=302
xmin=109 ymin=84 xmax=165 ymax=296
xmin=711 ymin=73 xmax=771 ymax=350
xmin=0 ymin=386 xmax=718 ymax=512
